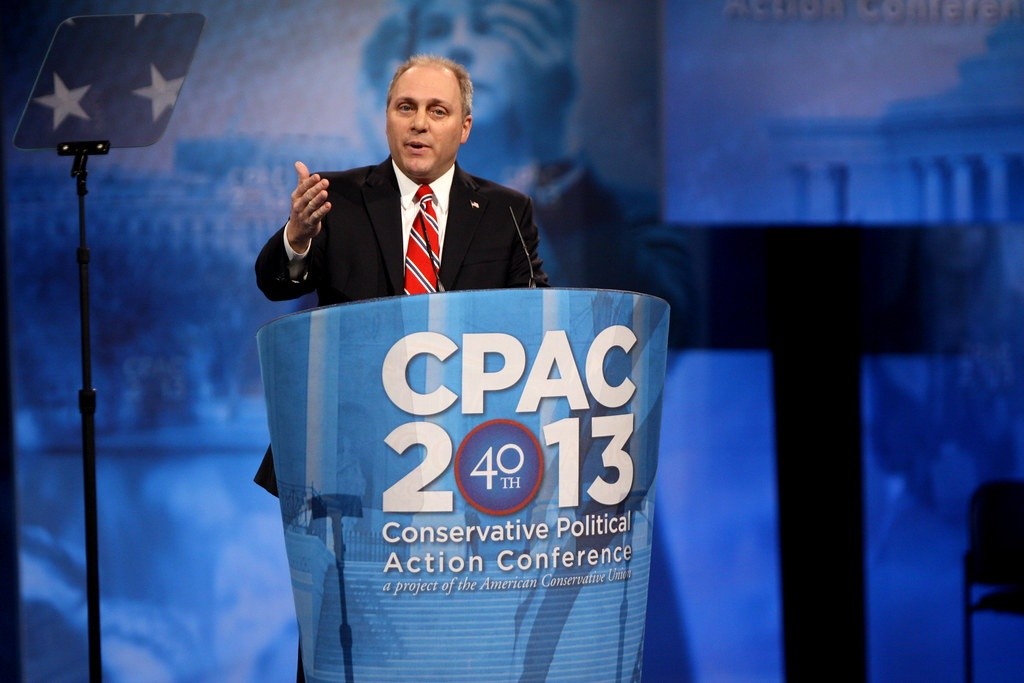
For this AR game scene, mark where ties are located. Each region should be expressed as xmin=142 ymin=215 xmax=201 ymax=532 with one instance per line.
xmin=402 ymin=183 xmax=440 ymax=296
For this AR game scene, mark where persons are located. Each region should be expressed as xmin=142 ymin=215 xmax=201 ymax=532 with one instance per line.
xmin=255 ymin=54 xmax=540 ymax=682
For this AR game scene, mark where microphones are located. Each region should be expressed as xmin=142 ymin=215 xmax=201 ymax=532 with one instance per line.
xmin=418 ymin=211 xmax=445 ymax=292
xmin=509 ymin=206 xmax=536 ymax=288
